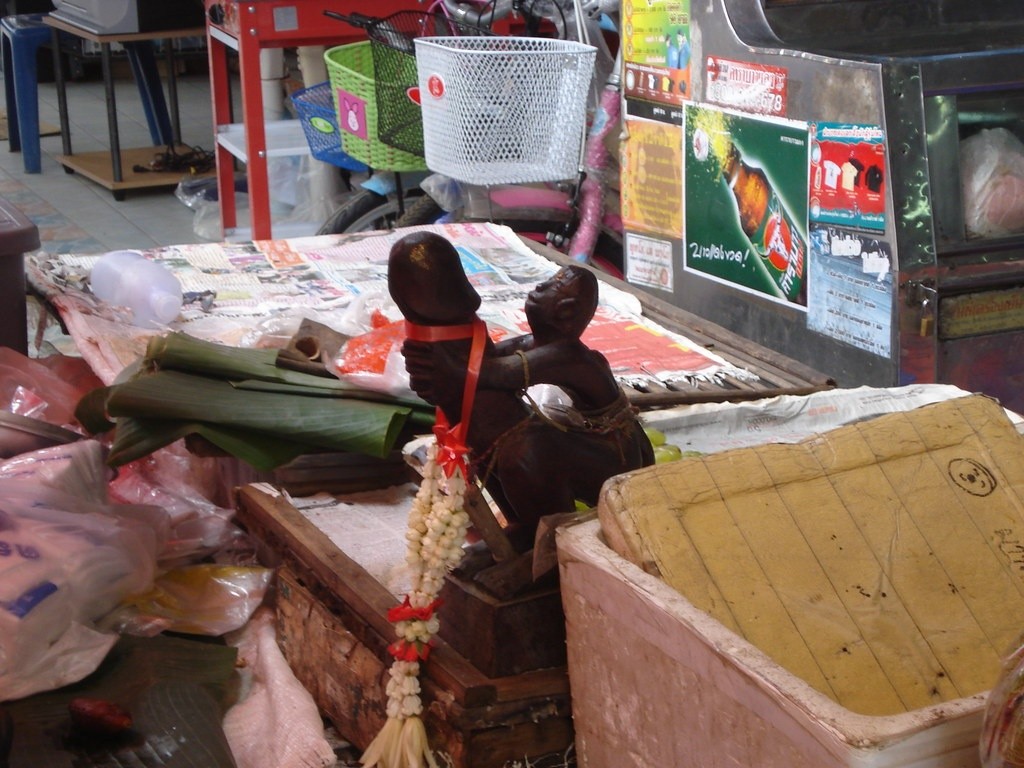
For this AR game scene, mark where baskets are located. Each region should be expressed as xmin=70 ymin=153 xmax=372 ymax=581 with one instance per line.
xmin=292 ymin=81 xmax=370 ymax=172
xmin=368 ymin=9 xmax=497 ymax=158
xmin=414 ymin=36 xmax=597 ymax=187
xmin=324 ymin=39 xmax=431 ymax=172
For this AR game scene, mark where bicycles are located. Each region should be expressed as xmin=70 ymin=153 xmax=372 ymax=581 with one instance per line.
xmin=290 ymin=0 xmax=626 ymax=281
